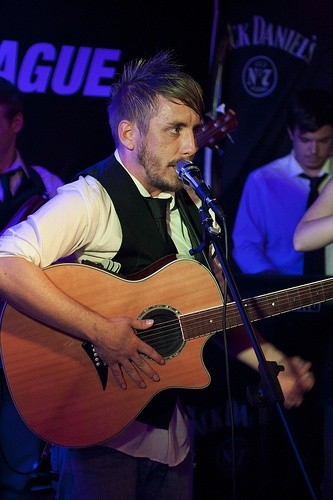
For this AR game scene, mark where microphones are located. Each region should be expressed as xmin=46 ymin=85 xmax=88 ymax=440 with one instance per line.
xmin=174 ymin=159 xmax=225 ymax=219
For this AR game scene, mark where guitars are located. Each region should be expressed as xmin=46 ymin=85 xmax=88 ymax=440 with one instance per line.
xmin=0 ymin=253 xmax=333 ymax=447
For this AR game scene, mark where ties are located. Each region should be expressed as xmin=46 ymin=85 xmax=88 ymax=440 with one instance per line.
xmin=143 ymin=197 xmax=171 ymax=250
xmin=1 ymin=171 xmax=15 ymax=200
xmin=299 ymin=173 xmax=330 ymax=283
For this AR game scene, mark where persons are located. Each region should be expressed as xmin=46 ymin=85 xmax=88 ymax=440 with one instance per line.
xmin=230 ymin=89 xmax=333 ymax=500
xmin=0 ymin=50 xmax=314 ymax=500
xmin=0 ymin=75 xmax=68 ymax=238
